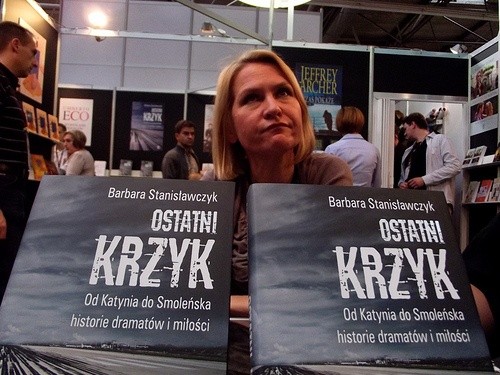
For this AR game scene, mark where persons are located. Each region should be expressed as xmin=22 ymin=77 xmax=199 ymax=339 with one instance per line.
xmin=203 ymin=49 xmax=353 ymax=318
xmin=326 ymin=106 xmax=382 ymax=187
xmin=162 ymin=120 xmax=203 ymax=181
xmin=474 ymin=72 xmax=487 ymax=97
xmin=54 ymin=123 xmax=66 ymax=171
xmin=398 ymin=112 xmax=462 ymax=216
xmin=394 ymin=125 xmax=410 ymax=188
xmin=463 ymin=213 xmax=500 ymax=329
xmin=0 ymin=21 xmax=39 ymax=299
xmin=60 ymin=131 xmax=72 ymax=174
xmin=474 ymin=102 xmax=487 ymax=121
xmin=65 ymin=130 xmax=96 ymax=176
xmin=485 ymin=101 xmax=496 ymax=116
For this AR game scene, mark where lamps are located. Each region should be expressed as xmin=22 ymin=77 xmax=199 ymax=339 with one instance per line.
xmin=450 ymin=44 xmax=462 ymax=53
xmin=201 ymin=21 xmax=227 ymax=38
xmin=90 ymin=22 xmax=106 ymax=43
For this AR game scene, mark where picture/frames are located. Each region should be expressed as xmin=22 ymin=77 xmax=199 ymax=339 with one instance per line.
xmin=15 ymin=17 xmax=47 ymax=108
xmin=48 ymin=114 xmax=60 ymax=143
xmin=30 ymin=154 xmax=48 ymax=180
xmin=35 ymin=108 xmax=48 ymax=137
xmin=21 ymin=101 xmax=37 ymax=133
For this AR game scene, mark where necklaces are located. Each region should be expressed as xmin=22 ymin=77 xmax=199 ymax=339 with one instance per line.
xmin=56 ymin=146 xmax=64 ymax=169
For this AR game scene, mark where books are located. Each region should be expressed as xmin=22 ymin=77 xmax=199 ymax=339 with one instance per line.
xmin=248 ymin=182 xmax=493 ymax=375
xmin=475 ymin=180 xmax=492 ymax=202
xmin=464 ymin=181 xmax=480 ymax=203
xmin=0 ymin=175 xmax=235 ymax=375
xmin=489 ymin=178 xmax=500 ymax=201
xmin=492 ymin=142 xmax=500 ymax=161
xmin=21 ymin=101 xmax=59 ymax=182
xmin=462 ymin=146 xmax=487 ymax=167
